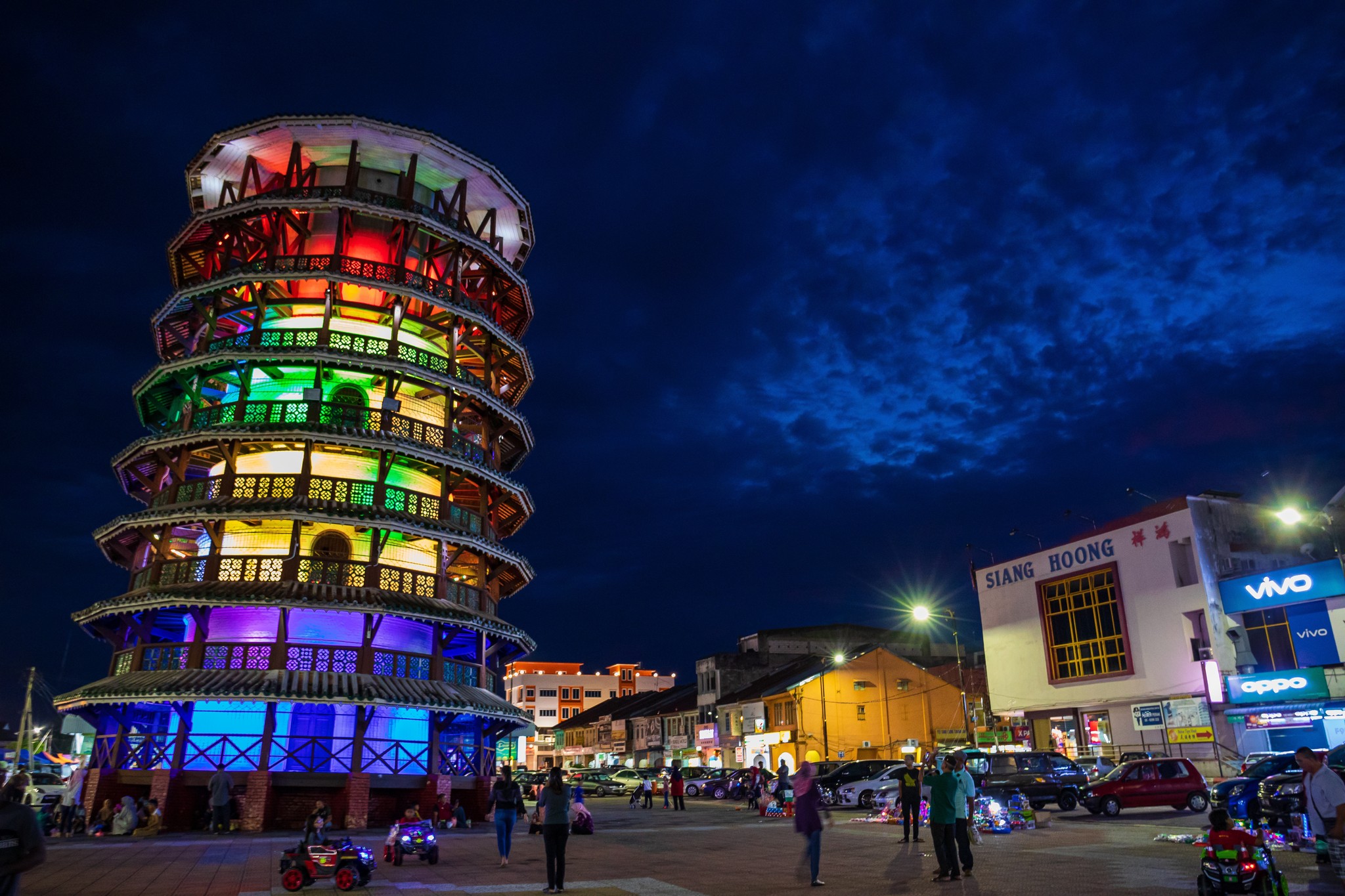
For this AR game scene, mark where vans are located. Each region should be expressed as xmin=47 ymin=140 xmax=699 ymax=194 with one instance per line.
xmin=965 ymin=751 xmax=1091 ymax=811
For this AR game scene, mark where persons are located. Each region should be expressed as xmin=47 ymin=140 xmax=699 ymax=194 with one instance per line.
xmin=399 ymin=801 xmax=425 ymax=824
xmin=50 ymin=768 xmax=84 ymax=837
xmin=0 ymin=781 xmax=45 ymax=896
xmin=1294 ymin=746 xmax=1345 ymax=886
xmin=487 ymin=765 xmax=528 ymax=867
xmin=432 ymin=793 xmax=472 ymax=829
xmin=918 ymin=753 xmax=962 ymax=882
xmin=204 ymin=763 xmax=234 ymax=835
xmin=776 ymin=759 xmax=789 ymax=807
xmin=529 ymin=802 xmax=546 ymax=834
xmin=0 ymin=760 xmax=30 ymax=805
xmin=50 ymin=796 xmax=163 ymax=840
xmin=745 ymin=761 xmax=768 ymax=811
xmin=570 ymin=802 xmax=594 ymax=835
xmin=668 ymin=766 xmax=687 ymax=811
xmin=641 ymin=774 xmax=653 ymax=809
xmin=790 ymin=759 xmax=834 ymax=886
xmin=662 ymin=776 xmax=669 ymax=809
xmin=303 ymin=799 xmax=333 ymax=833
xmin=929 ymin=743 xmax=975 ymax=877
xmin=528 ymin=784 xmax=544 ymax=801
xmin=898 ymin=754 xmax=925 ymax=842
xmin=1208 ymin=808 xmax=1264 ymax=861
xmin=539 ymin=766 xmax=572 ymax=894
xmin=572 ymin=777 xmax=589 ymax=804
xmin=1048 ymin=734 xmax=1055 ymax=752
xmin=305 ymin=813 xmax=338 ymax=853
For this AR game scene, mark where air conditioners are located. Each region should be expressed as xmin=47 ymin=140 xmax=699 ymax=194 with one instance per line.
xmin=907 ymin=738 xmax=918 ymax=747
xmin=1002 ymin=726 xmax=1010 ymax=731
xmin=1198 ymin=647 xmax=1213 ymax=660
xmin=863 ymin=740 xmax=870 ymax=747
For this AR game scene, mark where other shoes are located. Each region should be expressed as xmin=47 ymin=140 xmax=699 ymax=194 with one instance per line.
xmin=913 ymin=838 xmax=925 ymax=842
xmin=642 ymin=805 xmax=648 ymax=809
xmin=812 ymin=879 xmax=825 ymax=885
xmin=933 ymin=867 xmax=951 ymax=875
xmin=220 ymin=831 xmax=229 ymax=835
xmin=51 ymin=832 xmax=61 ymax=838
xmin=499 ymin=860 xmax=508 ymax=867
xmin=66 ymin=832 xmax=75 ymax=838
xmin=543 ymin=887 xmax=555 ymax=893
xmin=962 ymin=867 xmax=972 ymax=877
xmin=930 ymin=873 xmax=950 ymax=881
xmin=950 ymin=874 xmax=961 ymax=880
xmin=648 ymin=803 xmax=652 ymax=809
xmin=212 ymin=832 xmax=219 ymax=835
xmin=898 ymin=838 xmax=909 ymax=843
xmin=556 ymin=887 xmax=563 ymax=891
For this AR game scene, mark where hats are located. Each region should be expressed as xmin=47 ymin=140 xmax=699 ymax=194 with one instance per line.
xmin=953 ymin=749 xmax=967 ymax=761
xmin=945 ymin=754 xmax=957 ymax=766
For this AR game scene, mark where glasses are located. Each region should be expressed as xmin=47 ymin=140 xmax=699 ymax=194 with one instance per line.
xmin=314 ymin=818 xmax=324 ymax=824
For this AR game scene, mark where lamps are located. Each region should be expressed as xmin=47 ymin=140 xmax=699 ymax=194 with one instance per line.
xmin=381 ymin=397 xmax=401 ymax=413
xmin=303 ymin=387 xmax=323 ymax=401
xmin=1126 ymin=487 xmax=1157 ymax=504
xmin=966 ymin=543 xmax=994 ymax=563
xmin=1063 ymin=510 xmax=1097 ymax=531
xmin=1010 ymin=529 xmax=1042 ymax=550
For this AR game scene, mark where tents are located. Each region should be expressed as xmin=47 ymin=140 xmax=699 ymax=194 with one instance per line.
xmin=0 ymin=748 xmax=78 ymax=775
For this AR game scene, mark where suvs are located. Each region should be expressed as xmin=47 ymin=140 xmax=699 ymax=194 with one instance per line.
xmin=1256 ymin=739 xmax=1345 ymax=826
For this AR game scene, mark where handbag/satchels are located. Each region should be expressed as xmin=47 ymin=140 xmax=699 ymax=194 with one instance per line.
xmin=967 ymin=826 xmax=983 ymax=845
xmin=1323 ymin=817 xmax=1337 ymax=833
xmin=767 ymin=801 xmax=777 ymax=808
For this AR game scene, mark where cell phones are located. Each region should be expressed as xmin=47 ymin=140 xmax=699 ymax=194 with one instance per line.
xmin=925 ymin=752 xmax=929 ymax=762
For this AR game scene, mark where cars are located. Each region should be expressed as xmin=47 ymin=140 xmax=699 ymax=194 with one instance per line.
xmin=4 ymin=772 xmax=68 ymax=808
xmin=1241 ymin=751 xmax=1284 ymax=774
xmin=496 ymin=745 xmax=986 ymax=812
xmin=1071 ymin=756 xmax=1119 ymax=783
xmin=1119 ymin=751 xmax=1170 ymax=771
xmin=1209 ymin=748 xmax=1332 ymax=821
xmin=1078 ymin=756 xmax=1210 ymax=818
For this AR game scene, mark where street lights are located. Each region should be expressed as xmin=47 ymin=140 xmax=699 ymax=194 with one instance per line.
xmin=1279 ymin=506 xmax=1345 ymax=582
xmin=913 ymin=605 xmax=970 ymax=746
xmin=821 ymin=653 xmax=844 ymax=761
